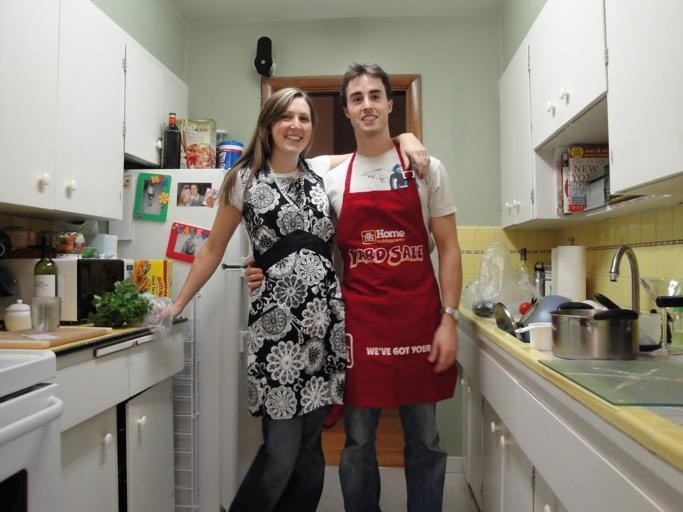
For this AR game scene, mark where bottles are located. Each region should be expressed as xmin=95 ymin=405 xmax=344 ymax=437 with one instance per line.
xmin=4 ymin=298 xmax=31 ymax=332
xmin=32 ymin=234 xmax=57 ymax=296
xmin=514 ymin=247 xmax=531 ymax=282
xmin=533 ymin=260 xmax=543 ymax=299
xmin=162 ymin=111 xmax=180 ymax=168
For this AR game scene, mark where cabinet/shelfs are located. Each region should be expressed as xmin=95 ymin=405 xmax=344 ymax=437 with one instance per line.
xmin=455 ymin=363 xmax=571 ymax=512
xmin=44 ymin=319 xmax=192 ymax=512
xmin=123 ymin=29 xmax=190 ymax=170
xmin=602 ymin=2 xmax=683 ymax=213
xmin=526 ymin=0 xmax=609 ymax=162
xmin=0 ymin=1 xmax=124 ymax=226
xmin=497 ymin=33 xmax=582 ymax=233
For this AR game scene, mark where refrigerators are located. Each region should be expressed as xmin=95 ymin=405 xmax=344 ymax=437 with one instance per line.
xmin=108 ymin=167 xmax=263 ymax=510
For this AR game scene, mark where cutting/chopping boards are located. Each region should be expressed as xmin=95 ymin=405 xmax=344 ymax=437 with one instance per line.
xmin=0 ymin=326 xmax=114 ymax=348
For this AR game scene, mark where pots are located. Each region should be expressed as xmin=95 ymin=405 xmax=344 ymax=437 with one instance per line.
xmin=549 ymin=293 xmax=640 ymax=360
xmin=516 ymin=294 xmax=569 ymax=343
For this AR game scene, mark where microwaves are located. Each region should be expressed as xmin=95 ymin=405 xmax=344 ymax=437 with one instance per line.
xmin=3 ymin=257 xmax=134 ymax=325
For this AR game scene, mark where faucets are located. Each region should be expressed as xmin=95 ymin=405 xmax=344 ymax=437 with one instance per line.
xmin=609 ymin=245 xmax=640 ymax=313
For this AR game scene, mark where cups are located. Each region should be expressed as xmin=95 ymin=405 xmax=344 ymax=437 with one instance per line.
xmin=28 ymin=295 xmax=62 ymax=330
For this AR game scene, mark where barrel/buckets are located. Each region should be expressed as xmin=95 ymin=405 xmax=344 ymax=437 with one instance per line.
xmin=216 ymin=141 xmax=243 ymax=169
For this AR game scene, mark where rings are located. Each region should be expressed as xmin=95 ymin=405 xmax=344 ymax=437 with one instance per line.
xmin=424 ymin=156 xmax=429 ymax=160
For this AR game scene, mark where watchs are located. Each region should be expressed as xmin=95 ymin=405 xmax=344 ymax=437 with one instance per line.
xmin=440 ymin=306 xmax=460 ymax=322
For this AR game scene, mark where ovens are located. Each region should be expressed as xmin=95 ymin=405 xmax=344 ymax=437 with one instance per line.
xmin=0 ymin=381 xmax=64 ymax=510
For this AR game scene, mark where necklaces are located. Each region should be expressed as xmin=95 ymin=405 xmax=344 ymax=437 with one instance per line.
xmin=267 ymin=159 xmax=307 ymax=213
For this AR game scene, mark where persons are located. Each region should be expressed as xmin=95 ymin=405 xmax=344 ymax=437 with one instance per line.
xmin=145 ymin=186 xmax=154 ymax=207
xmin=182 ymin=235 xmax=196 ymax=256
xmin=187 ymin=185 xmax=205 ymax=206
xmin=241 ymin=63 xmax=463 ymax=512
xmin=178 ymin=187 xmax=192 ymax=206
xmin=156 ymin=88 xmax=432 ymax=512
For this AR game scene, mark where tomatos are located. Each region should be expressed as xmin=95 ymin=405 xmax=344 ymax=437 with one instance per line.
xmin=520 ymin=303 xmax=532 ymax=314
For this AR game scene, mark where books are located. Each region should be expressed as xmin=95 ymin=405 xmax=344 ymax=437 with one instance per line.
xmin=553 ymin=141 xmax=614 ymax=215
xmin=133 ymin=259 xmax=174 ymax=304
